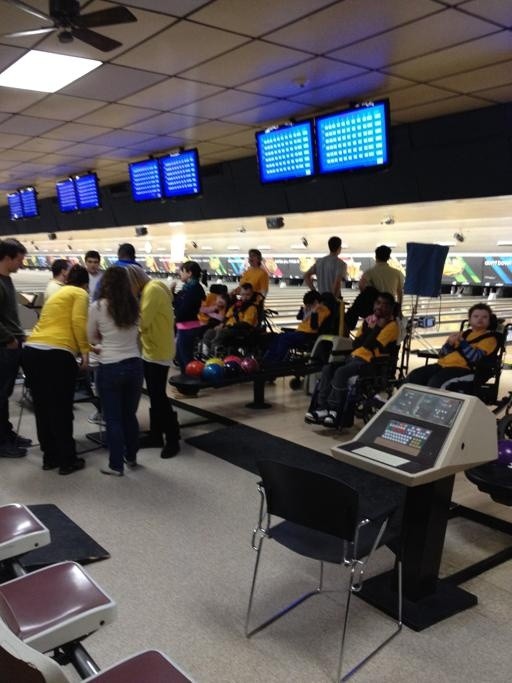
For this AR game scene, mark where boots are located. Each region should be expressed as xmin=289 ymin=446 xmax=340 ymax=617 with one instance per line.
xmin=139 ymin=408 xmax=164 ymax=448
xmin=161 ymin=412 xmax=181 ymax=459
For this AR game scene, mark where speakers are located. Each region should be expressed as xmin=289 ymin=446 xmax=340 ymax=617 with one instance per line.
xmin=136 ymin=227 xmax=147 ymax=236
xmin=266 ymin=217 xmax=284 ymax=229
xmin=48 ymin=234 xmax=56 ymax=239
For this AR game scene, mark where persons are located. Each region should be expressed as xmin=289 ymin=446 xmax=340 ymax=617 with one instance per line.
xmin=409 ymin=304 xmax=497 ymax=386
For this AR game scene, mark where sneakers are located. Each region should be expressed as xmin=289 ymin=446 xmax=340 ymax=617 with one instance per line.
xmin=0 ymin=443 xmax=27 ymax=458
xmin=1 ymin=430 xmax=31 ymax=446
xmin=59 ymin=458 xmax=86 ymax=474
xmin=99 ymin=465 xmax=123 ymax=476
xmin=42 ymin=455 xmax=61 ymax=470
xmin=88 ymin=411 xmax=107 ymax=425
xmin=123 ymin=456 xmax=136 ymax=469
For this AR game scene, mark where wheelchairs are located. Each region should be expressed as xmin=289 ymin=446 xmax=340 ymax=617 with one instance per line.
xmin=280 ymin=291 xmax=351 ymax=390
xmin=174 ymin=290 xmax=238 ymax=365
xmin=196 ymin=289 xmax=279 ymax=361
xmin=491 ymin=396 xmax=512 ymax=444
xmin=417 ymin=317 xmax=512 ymax=404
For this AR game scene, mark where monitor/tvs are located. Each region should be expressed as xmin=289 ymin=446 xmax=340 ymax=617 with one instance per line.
xmin=20 ymin=187 xmax=40 ymax=217
xmin=56 ymin=179 xmax=79 ymax=213
xmin=128 ymin=158 xmax=162 ymax=203
xmin=315 ymin=98 xmax=391 ymax=175
xmin=74 ymin=172 xmax=101 ymax=211
xmin=255 ymin=118 xmax=317 ymax=186
xmin=159 ymin=148 xmax=203 ymax=200
xmin=8 ymin=191 xmax=24 ymax=221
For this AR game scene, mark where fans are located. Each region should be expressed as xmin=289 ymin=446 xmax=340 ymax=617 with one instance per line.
xmin=0 ymin=0 xmax=139 ymax=54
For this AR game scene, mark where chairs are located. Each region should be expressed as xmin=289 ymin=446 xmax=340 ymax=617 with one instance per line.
xmin=243 ymin=458 xmax=404 ymax=683
xmin=408 ymin=315 xmax=512 ymax=416
xmin=193 ymin=292 xmax=351 ymax=390
xmin=1 ymin=560 xmax=118 ymax=653
xmin=1 ymin=501 xmax=51 ymax=561
xmin=0 ymin=617 xmax=196 ymax=683
